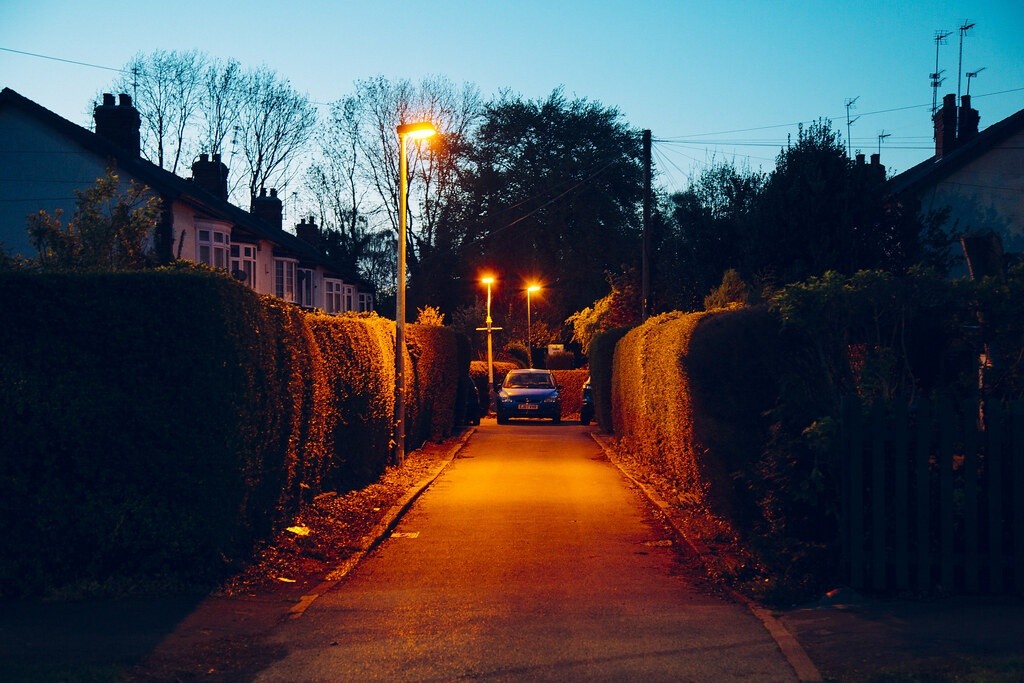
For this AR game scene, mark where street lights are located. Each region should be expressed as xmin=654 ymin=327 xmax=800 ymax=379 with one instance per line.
xmin=527 ymin=280 xmax=542 ymax=369
xmin=480 ymin=274 xmax=497 ymax=412
xmin=398 ymin=122 xmax=436 ymax=463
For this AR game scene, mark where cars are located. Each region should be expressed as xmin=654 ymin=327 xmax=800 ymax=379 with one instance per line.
xmin=579 ymin=376 xmax=598 ymax=425
xmin=469 ymin=377 xmax=481 ymax=425
xmin=495 ymin=367 xmax=563 ymax=425
